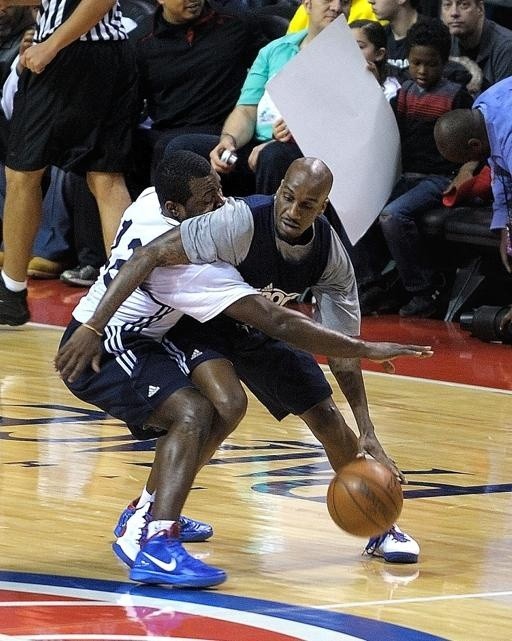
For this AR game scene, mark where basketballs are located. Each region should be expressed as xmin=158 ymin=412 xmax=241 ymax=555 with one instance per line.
xmin=327 ymin=460 xmax=403 ymax=539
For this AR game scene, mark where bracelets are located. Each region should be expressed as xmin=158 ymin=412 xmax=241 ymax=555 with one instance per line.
xmin=84 ymin=327 xmax=104 ymax=335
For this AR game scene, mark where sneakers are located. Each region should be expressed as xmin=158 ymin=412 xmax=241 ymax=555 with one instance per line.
xmin=112 ymin=493 xmax=228 ymax=589
xmin=399 ymin=278 xmax=456 ymax=319
xmin=363 ymin=517 xmax=422 ymax=565
xmin=0 ymin=250 xmax=101 ymax=328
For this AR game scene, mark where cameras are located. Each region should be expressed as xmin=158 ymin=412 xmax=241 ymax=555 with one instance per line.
xmin=471 ymin=306 xmax=512 ymax=344
xmin=220 ymin=149 xmax=238 ymax=166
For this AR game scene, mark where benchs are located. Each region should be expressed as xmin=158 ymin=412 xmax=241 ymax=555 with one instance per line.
xmin=445 ymin=209 xmax=512 ymax=322
xmin=422 ymin=207 xmax=479 ymax=247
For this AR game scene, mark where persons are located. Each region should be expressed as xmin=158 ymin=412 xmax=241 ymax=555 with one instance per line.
xmin=1 ymin=1 xmax=512 ymax=325
